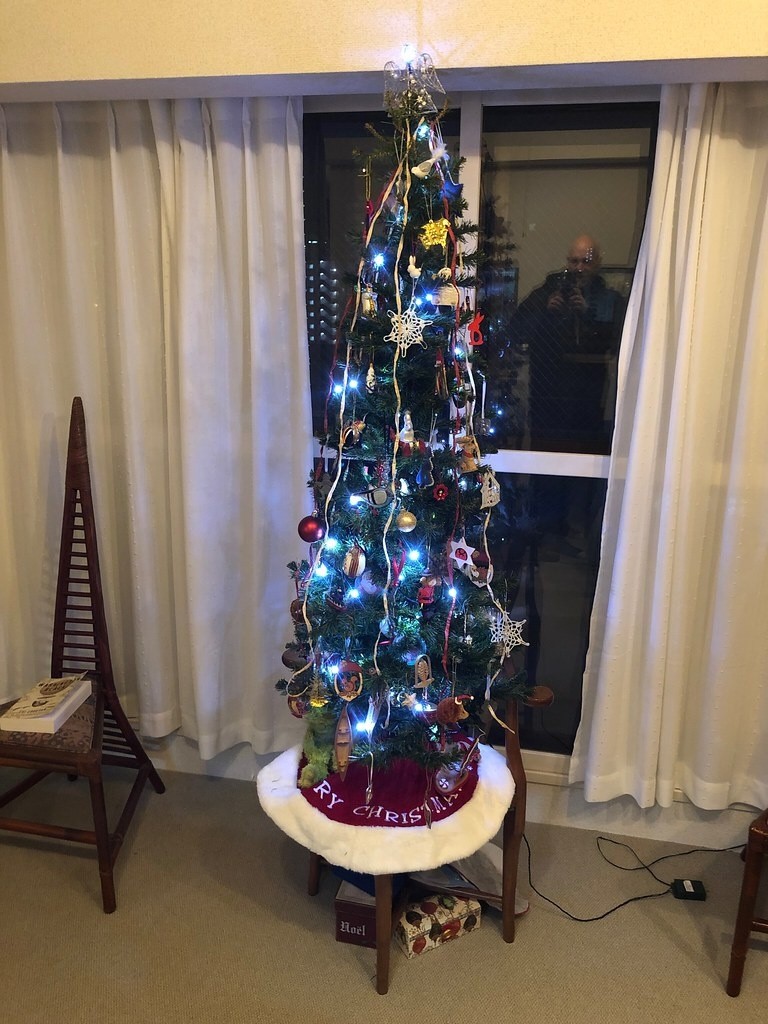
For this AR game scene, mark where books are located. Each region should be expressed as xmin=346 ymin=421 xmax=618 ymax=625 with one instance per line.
xmin=1 ymin=669 xmax=92 ymax=733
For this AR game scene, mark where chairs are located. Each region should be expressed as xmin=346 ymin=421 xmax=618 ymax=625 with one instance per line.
xmin=300 ymin=688 xmax=537 ymax=994
xmin=0 ymin=393 xmax=167 ymax=913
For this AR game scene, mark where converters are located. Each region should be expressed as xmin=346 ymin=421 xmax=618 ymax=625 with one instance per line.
xmin=670 ymin=879 xmax=707 ymax=901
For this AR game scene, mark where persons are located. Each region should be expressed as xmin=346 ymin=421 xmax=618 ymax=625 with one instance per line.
xmin=499 ymin=234 xmax=630 ymax=560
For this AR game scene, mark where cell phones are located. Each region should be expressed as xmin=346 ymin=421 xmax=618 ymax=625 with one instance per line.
xmin=559 ymin=272 xmax=576 ymax=303
